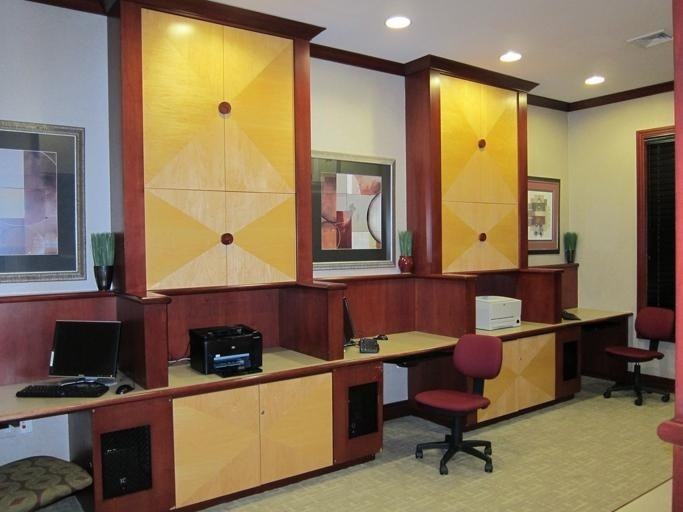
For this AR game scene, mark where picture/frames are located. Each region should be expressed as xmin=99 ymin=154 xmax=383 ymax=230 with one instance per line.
xmin=527 ymin=175 xmax=561 ymax=255
xmin=0 ymin=119 xmax=87 ymax=283
xmin=312 ymin=150 xmax=397 ymax=272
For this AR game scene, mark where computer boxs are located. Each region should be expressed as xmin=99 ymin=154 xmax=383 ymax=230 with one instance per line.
xmin=563 ymin=341 xmax=577 ymax=385
xmin=101 ymin=427 xmax=146 ymax=497
xmin=350 ymin=386 xmax=375 ymax=436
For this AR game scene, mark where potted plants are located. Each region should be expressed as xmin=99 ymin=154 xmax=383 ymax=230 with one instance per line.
xmin=398 ymin=230 xmax=414 ymax=273
xmin=90 ymin=232 xmax=116 ymax=293
xmin=563 ymin=232 xmax=577 ymax=263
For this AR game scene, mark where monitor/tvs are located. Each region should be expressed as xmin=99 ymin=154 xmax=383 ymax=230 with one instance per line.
xmin=343 ymin=297 xmax=360 ymax=347
xmin=49 ymin=319 xmax=122 ymax=386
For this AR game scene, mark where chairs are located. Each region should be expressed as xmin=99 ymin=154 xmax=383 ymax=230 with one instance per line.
xmin=414 ymin=334 xmax=503 ymax=474
xmin=603 ymin=307 xmax=675 ymax=405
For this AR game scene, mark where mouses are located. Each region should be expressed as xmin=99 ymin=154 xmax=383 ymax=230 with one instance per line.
xmin=116 ymin=384 xmax=133 ymax=394
xmin=378 ymin=334 xmax=388 ymax=340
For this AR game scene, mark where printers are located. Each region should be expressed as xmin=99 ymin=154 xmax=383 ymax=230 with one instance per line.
xmin=189 ymin=323 xmax=263 ymax=378
xmin=475 ymin=295 xmax=523 ymax=330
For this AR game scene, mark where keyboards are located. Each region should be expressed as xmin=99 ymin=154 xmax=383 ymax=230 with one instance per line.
xmin=563 ymin=313 xmax=579 ymax=320
xmin=360 ymin=337 xmax=379 ymax=353
xmin=16 ymin=384 xmax=109 ymax=397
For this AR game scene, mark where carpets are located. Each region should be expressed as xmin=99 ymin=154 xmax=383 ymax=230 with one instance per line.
xmin=225 ymin=388 xmax=676 ymax=512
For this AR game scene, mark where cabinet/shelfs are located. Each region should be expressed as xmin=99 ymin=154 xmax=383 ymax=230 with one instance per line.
xmin=108 ymin=0 xmax=327 ymax=293
xmin=173 ymin=371 xmax=333 ymax=511
xmin=554 ymin=324 xmax=584 ymax=403
xmin=477 ymin=334 xmax=556 ymax=423
xmin=67 ymin=394 xmax=176 ymax=512
xmin=333 ymin=360 xmax=383 ymax=472
xmin=404 ymin=55 xmax=539 ymax=274
